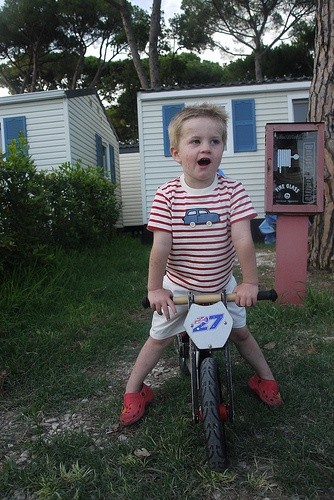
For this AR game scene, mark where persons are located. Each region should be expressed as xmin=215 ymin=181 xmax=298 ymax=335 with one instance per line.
xmin=120 ymin=104 xmax=284 ymax=426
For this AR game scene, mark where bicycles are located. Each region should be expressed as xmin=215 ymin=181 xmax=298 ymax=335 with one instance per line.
xmin=140 ymin=287 xmax=278 ymax=473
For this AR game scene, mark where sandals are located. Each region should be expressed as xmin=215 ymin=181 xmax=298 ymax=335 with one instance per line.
xmin=247 ymin=372 xmax=284 ymax=407
xmin=119 ymin=385 xmax=154 ymax=426
